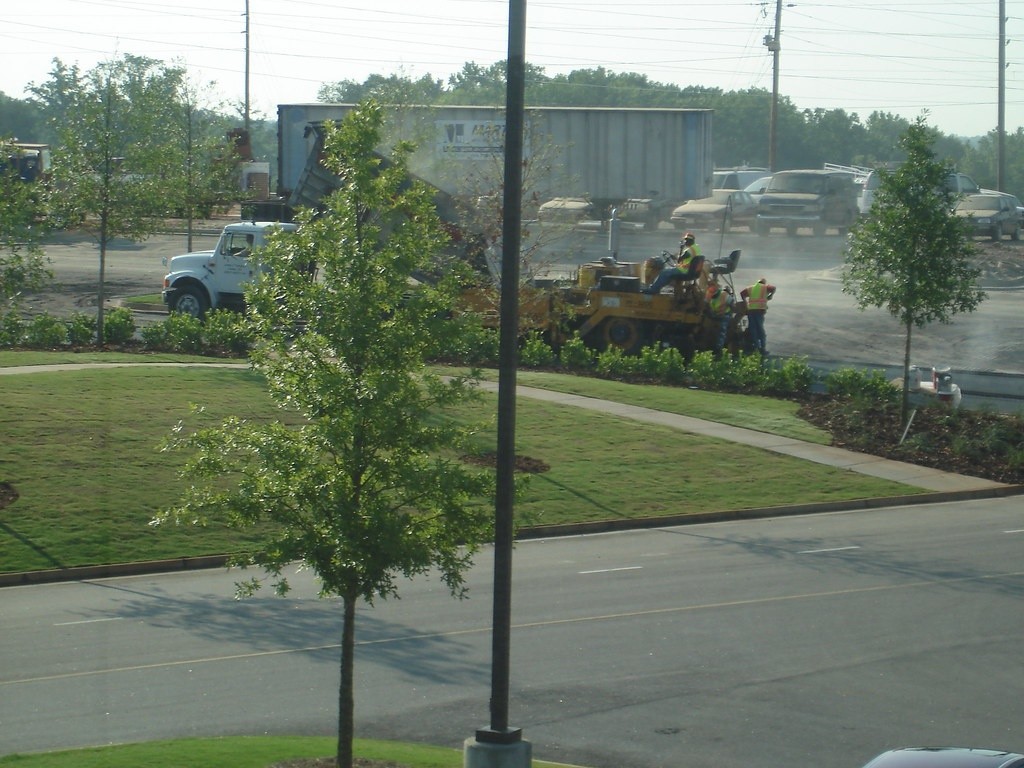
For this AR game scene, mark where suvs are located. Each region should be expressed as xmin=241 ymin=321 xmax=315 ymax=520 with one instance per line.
xmin=855 ymin=168 xmax=910 ymax=224
xmin=756 ymin=171 xmax=853 ymax=239
xmin=938 ymin=173 xmax=981 ymax=195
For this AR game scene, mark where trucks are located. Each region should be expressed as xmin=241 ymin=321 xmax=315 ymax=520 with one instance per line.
xmin=158 ymin=125 xmax=519 ymax=322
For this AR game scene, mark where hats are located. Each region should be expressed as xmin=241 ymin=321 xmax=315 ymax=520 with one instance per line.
xmin=683 ymin=232 xmax=695 ymax=240
xmin=707 ymin=287 xmax=719 ymax=297
xmin=758 ymin=278 xmax=766 ymax=284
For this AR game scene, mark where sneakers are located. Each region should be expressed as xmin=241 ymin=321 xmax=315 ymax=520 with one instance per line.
xmin=642 ymin=287 xmax=660 ymax=296
xmin=759 ymin=349 xmax=770 ymax=355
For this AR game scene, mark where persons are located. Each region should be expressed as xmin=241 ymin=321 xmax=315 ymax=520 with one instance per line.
xmin=643 ymin=232 xmax=776 ymax=359
xmin=233 ymin=234 xmax=254 ymax=258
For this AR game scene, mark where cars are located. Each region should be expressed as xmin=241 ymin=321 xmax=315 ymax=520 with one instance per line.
xmin=949 ymin=192 xmax=1020 ymax=241
xmin=671 ymin=189 xmax=755 ymax=234
xmin=712 ymin=172 xmax=771 ymax=199
xmin=742 ymin=175 xmax=773 ymax=205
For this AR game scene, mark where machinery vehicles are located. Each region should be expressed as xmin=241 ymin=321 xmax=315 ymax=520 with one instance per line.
xmin=522 ymin=241 xmax=768 ymax=362
